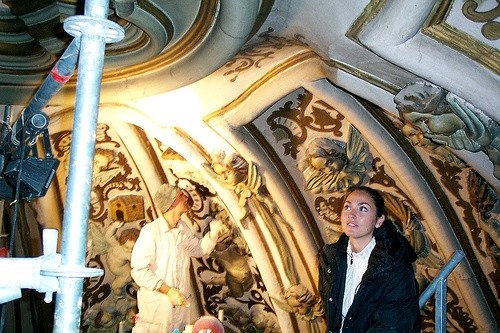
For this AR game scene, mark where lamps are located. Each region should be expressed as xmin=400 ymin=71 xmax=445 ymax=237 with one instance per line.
xmin=0 ymin=129 xmax=61 ymax=202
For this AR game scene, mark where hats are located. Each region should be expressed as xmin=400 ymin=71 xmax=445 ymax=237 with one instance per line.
xmin=153 ymin=183 xmax=180 ymax=214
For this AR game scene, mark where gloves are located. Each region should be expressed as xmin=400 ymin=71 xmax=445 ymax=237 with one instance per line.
xmin=166 ymin=286 xmax=189 ymax=308
xmin=208 ymin=218 xmax=230 ymax=240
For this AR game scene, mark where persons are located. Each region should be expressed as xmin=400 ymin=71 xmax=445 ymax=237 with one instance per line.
xmin=0 ymin=253 xmax=61 ymax=304
xmin=132 ymin=183 xmax=222 ymax=333
xmin=318 ymin=186 xmax=422 ymax=333
